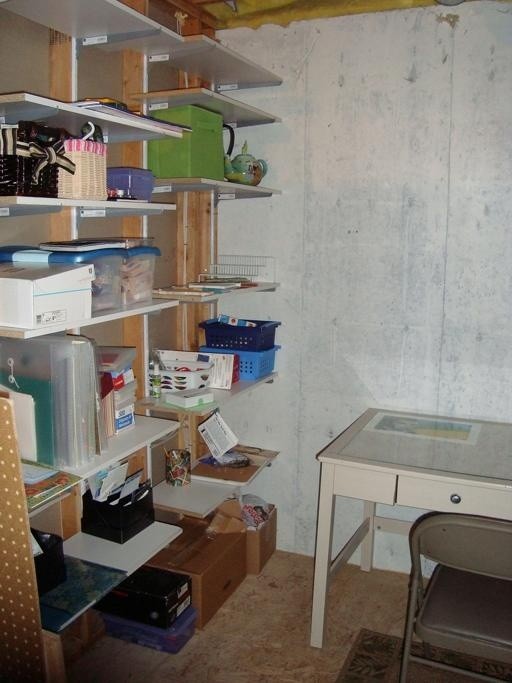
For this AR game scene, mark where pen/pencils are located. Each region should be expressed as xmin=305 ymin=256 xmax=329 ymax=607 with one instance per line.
xmin=164 ymin=448 xmax=189 ymax=458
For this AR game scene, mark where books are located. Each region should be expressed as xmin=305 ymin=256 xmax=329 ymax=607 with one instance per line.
xmin=156 ymin=276 xmax=257 ymax=298
xmin=79 ymin=103 xmax=184 ymax=132
xmin=20 ymin=460 xmax=84 ymax=510
xmin=62 ymin=99 xmax=194 ymax=132
xmin=39 ymin=239 xmax=126 ymax=251
xmin=107 ymin=197 xmax=149 ymax=202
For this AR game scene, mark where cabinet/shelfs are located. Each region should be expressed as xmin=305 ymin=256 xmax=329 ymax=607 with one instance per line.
xmin=0 ymin=1 xmax=279 ymax=631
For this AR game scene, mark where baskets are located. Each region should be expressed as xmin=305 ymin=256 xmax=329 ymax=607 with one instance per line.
xmin=198 ymin=318 xmax=281 ymax=352
xmin=148 ymin=360 xmax=214 ymax=394
xmin=198 ymin=346 xmax=281 ymax=381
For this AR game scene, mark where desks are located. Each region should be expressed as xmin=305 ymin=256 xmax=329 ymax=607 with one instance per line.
xmin=310 ymin=408 xmax=512 ymax=650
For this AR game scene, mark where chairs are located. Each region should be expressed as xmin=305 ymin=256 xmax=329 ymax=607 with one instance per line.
xmin=399 ymin=511 xmax=512 ymax=683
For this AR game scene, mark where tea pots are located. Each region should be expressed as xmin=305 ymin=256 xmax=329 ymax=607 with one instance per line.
xmin=223 ymin=139 xmax=267 ymax=186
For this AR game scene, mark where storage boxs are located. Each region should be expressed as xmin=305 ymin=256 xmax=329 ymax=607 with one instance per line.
xmin=98 ymin=499 xmax=277 ymax=654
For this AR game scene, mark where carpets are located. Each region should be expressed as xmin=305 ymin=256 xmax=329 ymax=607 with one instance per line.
xmin=335 ymin=629 xmax=512 ymax=683
